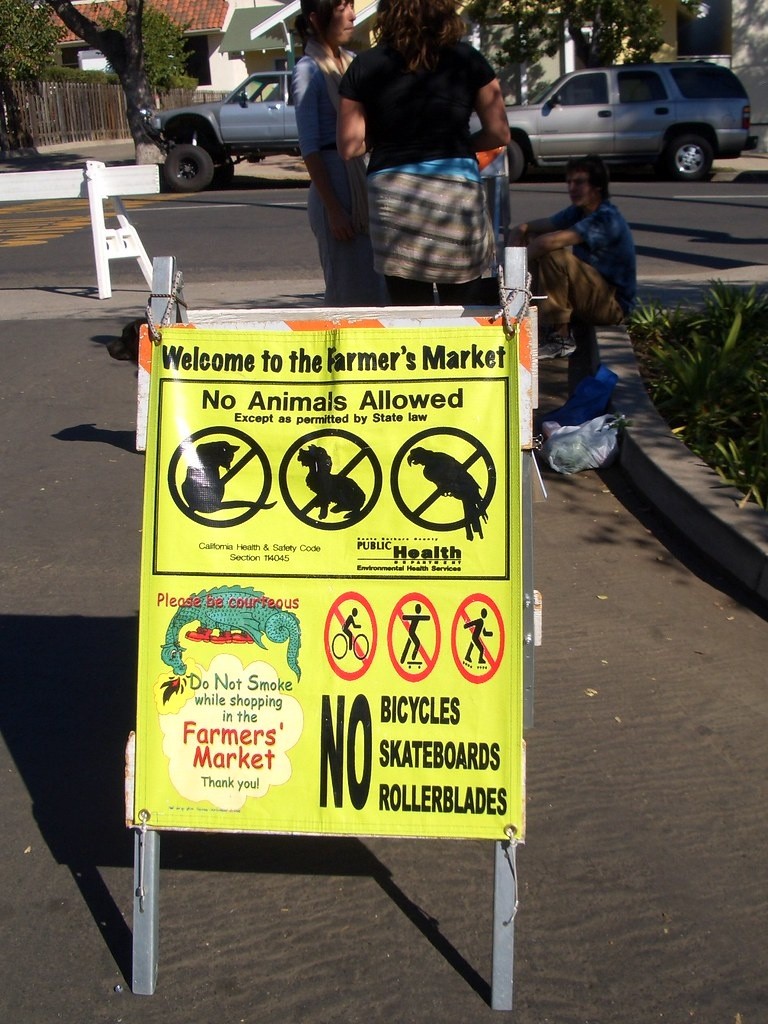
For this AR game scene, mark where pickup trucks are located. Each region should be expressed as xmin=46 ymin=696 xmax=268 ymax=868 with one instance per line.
xmin=150 ymin=71 xmax=300 ymax=194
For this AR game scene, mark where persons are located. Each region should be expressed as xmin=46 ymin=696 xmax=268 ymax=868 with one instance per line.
xmin=289 ymin=0 xmax=393 ymax=307
xmin=335 ymin=0 xmax=511 ymax=306
xmin=507 ymin=153 xmax=637 ymax=360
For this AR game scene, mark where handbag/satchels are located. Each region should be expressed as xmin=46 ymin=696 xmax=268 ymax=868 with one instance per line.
xmin=539 ymin=413 xmax=621 ymax=476
xmin=537 ymin=364 xmax=618 ymax=431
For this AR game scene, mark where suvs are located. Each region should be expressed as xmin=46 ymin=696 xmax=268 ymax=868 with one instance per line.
xmin=469 ymin=59 xmax=752 ymax=183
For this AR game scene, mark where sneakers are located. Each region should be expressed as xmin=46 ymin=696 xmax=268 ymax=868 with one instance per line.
xmin=539 ymin=330 xmax=577 ymax=359
